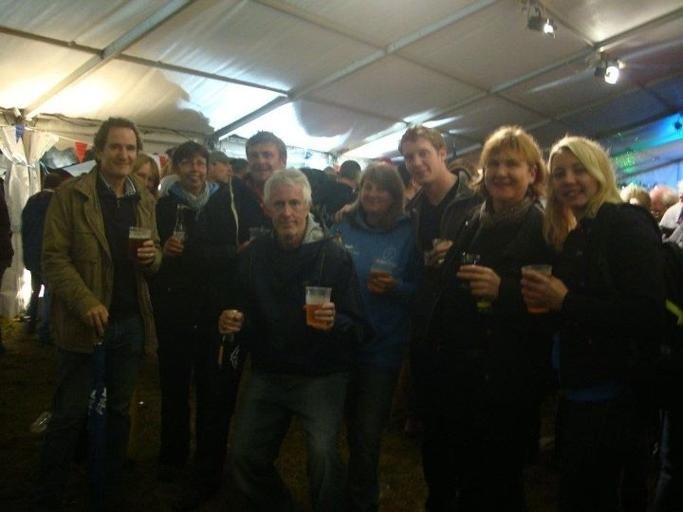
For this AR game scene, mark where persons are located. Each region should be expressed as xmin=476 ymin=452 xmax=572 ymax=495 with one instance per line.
xmin=132 ymin=150 xmax=161 ymax=199
xmin=39 ymin=117 xmax=164 ymax=511
xmin=232 ymin=157 xmax=248 ymax=177
xmin=449 ymin=126 xmax=555 ymax=512
xmin=524 ymin=137 xmax=666 ymax=509
xmin=208 ymin=152 xmax=231 ymax=184
xmin=649 ymin=184 xmax=678 ymax=223
xmin=156 ymin=140 xmax=239 ymax=510
xmin=399 ymin=126 xmax=484 ymax=511
xmin=240 ymin=131 xmax=286 ymax=220
xmin=217 ymin=168 xmax=369 ymax=512
xmin=327 ymin=159 xmax=363 ymax=214
xmin=21 ymin=173 xmax=63 ymax=316
xmin=330 ymin=160 xmax=420 ymax=511
xmin=619 ymin=184 xmax=650 ymax=210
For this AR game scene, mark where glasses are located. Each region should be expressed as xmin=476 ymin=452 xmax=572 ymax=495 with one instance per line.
xmin=594 ymin=55 xmax=625 ymax=87
xmin=525 ymin=14 xmax=555 ymax=36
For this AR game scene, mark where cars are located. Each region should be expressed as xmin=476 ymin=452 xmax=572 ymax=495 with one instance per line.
xmin=305 ymin=285 xmax=333 ymax=327
xmin=128 ymin=226 xmax=152 ymax=267
xmin=248 ymin=225 xmax=271 ymax=244
xmin=524 ymin=263 xmax=553 ymax=314
xmin=432 ymin=237 xmax=449 ymax=267
xmin=461 ymin=251 xmax=482 ymax=266
xmin=369 ymin=257 xmax=398 ymax=295
